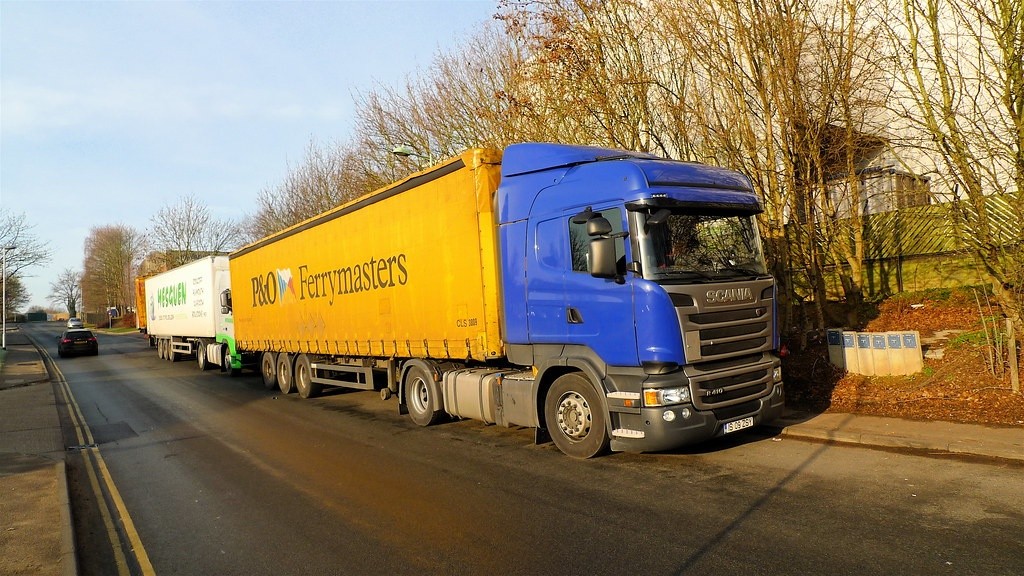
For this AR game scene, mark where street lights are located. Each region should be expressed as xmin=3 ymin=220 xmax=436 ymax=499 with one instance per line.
xmin=97 ymin=259 xmax=112 ymax=329
xmin=2 ymin=245 xmax=17 ymax=351
xmin=391 ymin=148 xmax=433 ymax=168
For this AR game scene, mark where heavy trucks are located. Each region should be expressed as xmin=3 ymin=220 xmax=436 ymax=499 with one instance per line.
xmin=144 ymin=255 xmax=261 ymax=377
xmin=227 ymin=142 xmax=788 ymax=459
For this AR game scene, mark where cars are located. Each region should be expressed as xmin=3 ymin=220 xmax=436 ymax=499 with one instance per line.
xmin=55 ymin=328 xmax=100 ymax=357
xmin=67 ymin=318 xmax=83 ymax=329
